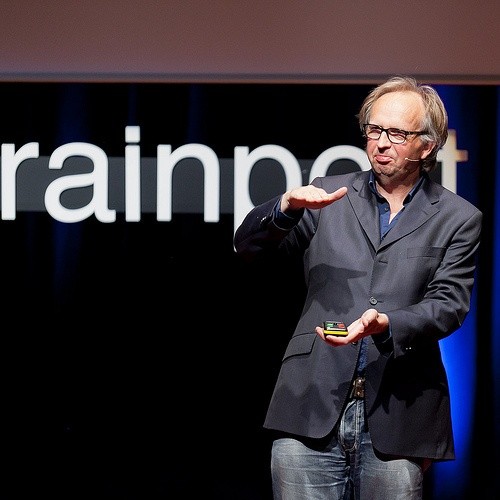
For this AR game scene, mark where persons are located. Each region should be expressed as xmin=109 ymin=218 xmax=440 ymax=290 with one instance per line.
xmin=234 ymin=75 xmax=483 ymax=500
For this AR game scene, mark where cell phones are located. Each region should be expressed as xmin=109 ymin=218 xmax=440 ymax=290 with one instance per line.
xmin=323 ymin=321 xmax=348 ymax=336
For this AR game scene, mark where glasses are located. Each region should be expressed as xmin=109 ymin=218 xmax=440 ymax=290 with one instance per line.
xmin=362 ymin=123 xmax=428 ymax=144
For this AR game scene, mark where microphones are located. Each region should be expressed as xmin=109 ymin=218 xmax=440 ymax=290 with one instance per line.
xmin=405 ymin=158 xmax=421 ymax=162
